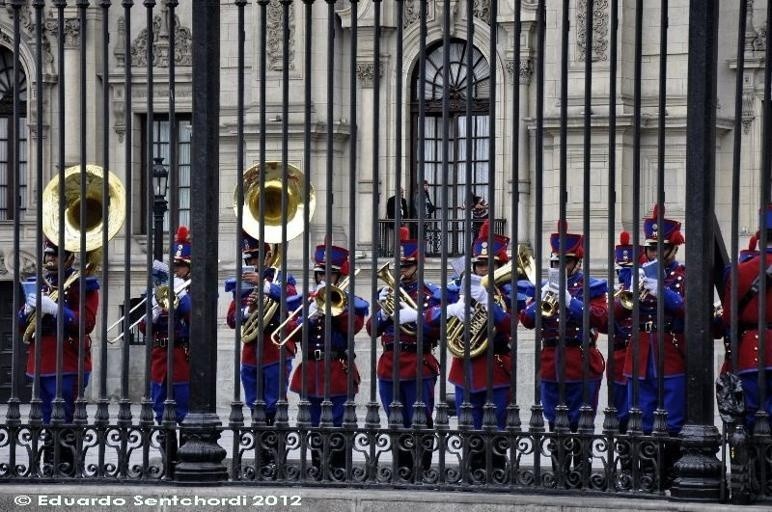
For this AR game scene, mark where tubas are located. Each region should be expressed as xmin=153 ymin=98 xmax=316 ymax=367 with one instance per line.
xmin=234 ymin=162 xmax=316 ymax=343
xmin=23 ymin=165 xmax=126 ymax=344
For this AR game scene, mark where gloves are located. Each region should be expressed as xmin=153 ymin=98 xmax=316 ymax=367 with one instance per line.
xmin=23 ymin=293 xmax=58 ymax=316
xmin=541 ymin=282 xmax=571 ymax=308
xmin=447 ymin=296 xmax=474 ymax=322
xmin=630 ymin=268 xmax=657 ymax=296
xmin=309 ymin=280 xmax=333 ymax=319
xmin=471 ymin=284 xmax=488 ymax=311
xmin=379 ymin=286 xmax=389 ymax=321
xmin=152 ymin=278 xmax=186 ymax=311
xmin=391 ymin=302 xmax=417 ymax=324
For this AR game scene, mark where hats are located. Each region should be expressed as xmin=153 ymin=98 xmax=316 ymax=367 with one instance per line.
xmin=550 ymin=232 xmax=584 ymax=261
xmin=644 ymin=219 xmax=681 ymax=247
xmin=615 ymin=245 xmax=645 ymax=269
xmin=759 ymin=204 xmax=772 ymax=229
xmin=44 ymin=238 xmax=68 ymax=256
xmin=393 ymin=240 xmax=427 ymax=266
xmin=313 ymin=244 xmax=349 ymax=275
xmin=242 ymin=233 xmax=276 ymax=259
xmin=169 ymin=240 xmax=190 ymax=263
xmin=472 ymin=232 xmax=509 ymax=260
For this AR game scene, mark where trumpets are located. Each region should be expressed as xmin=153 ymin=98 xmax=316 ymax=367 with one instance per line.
xmin=540 ymin=293 xmax=558 ymax=318
xmin=612 ymin=277 xmax=652 ymax=309
xmin=376 ymin=263 xmax=419 ymax=335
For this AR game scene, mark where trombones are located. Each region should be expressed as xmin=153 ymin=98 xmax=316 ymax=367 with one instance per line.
xmin=105 ymin=275 xmax=191 ymax=344
xmin=271 ymin=268 xmax=359 ymax=346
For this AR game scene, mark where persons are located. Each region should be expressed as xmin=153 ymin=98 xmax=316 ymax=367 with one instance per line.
xmin=471 ymin=194 xmax=487 ymax=255
xmin=225 ymin=230 xmax=301 ymax=478
xmin=280 ymin=240 xmax=370 ymax=480
xmin=604 ymin=243 xmax=646 ymax=487
xmin=612 ymin=217 xmax=691 ymax=491
xmin=17 ymin=236 xmax=101 ymax=475
xmin=718 ymin=202 xmax=772 ymax=498
xmin=721 ymin=248 xmax=760 ymax=374
xmin=139 ymin=225 xmax=197 ymax=477
xmin=363 ymin=239 xmax=445 ymax=480
xmin=518 ymin=231 xmax=612 ymax=486
xmin=425 ymin=231 xmax=525 ymax=483
xmin=410 ymin=178 xmax=436 ymax=257
xmin=386 ymin=186 xmax=407 ymax=257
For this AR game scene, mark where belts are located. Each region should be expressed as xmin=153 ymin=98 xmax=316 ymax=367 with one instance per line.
xmin=384 ymin=342 xmax=431 ymax=353
xmin=740 ymin=323 xmax=772 ymax=329
xmin=543 ymin=336 xmax=582 ymax=346
xmin=614 ymin=340 xmax=629 ymax=350
xmin=41 ymin=327 xmax=73 ymax=337
xmin=308 ymin=350 xmax=348 ymax=360
xmin=639 ymin=321 xmax=672 ymax=333
xmin=152 ymin=337 xmax=188 ymax=347
xmin=499 ymin=339 xmax=511 ymax=355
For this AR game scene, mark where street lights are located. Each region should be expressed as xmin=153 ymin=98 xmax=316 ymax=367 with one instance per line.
xmin=149 ymin=156 xmax=170 ymax=265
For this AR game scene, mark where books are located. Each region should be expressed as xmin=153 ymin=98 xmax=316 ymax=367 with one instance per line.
xmin=20 ymin=280 xmax=38 ymax=303
xmin=240 ymin=265 xmax=256 ymax=290
xmin=547 ymin=266 xmax=569 ymax=292
xmin=458 ymin=273 xmax=483 ymax=297
xmin=642 ymin=259 xmax=669 ymax=282
